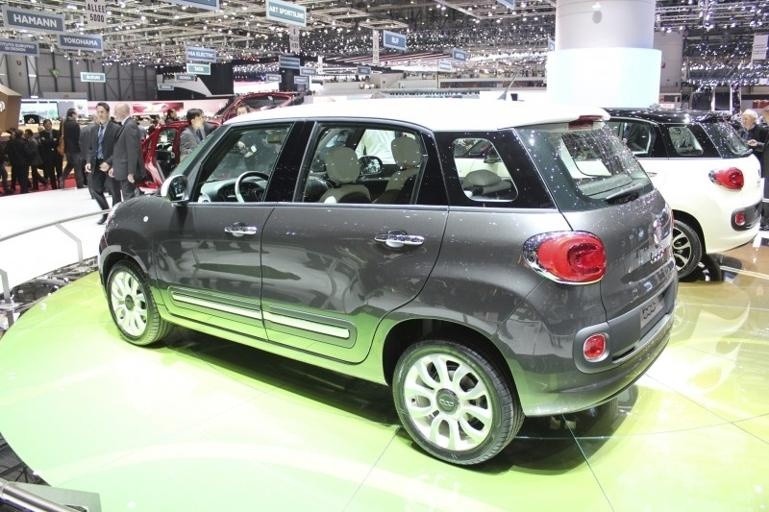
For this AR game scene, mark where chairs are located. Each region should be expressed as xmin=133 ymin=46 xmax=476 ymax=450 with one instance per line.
xmin=319 ymin=136 xmax=421 ymax=204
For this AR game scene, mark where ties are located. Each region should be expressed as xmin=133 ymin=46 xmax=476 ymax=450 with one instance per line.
xmin=97 ymin=126 xmax=104 ymax=159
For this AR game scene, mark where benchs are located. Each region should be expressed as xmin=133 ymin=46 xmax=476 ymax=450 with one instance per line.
xmin=461 ymin=169 xmax=515 ymax=198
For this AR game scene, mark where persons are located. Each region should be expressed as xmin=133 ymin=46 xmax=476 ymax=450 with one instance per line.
xmin=737 ymin=108 xmax=768 ymax=176
xmin=0 ymin=108 xmax=96 ymax=196
xmin=107 ymin=102 xmax=147 ymax=202
xmin=197 ymin=112 xmax=218 ymax=141
xmin=179 ymin=108 xmax=203 ymax=166
xmin=746 ymin=104 xmax=769 ymax=230
xmin=85 ymin=102 xmax=122 ymax=225
xmin=131 ymin=105 xmax=180 ymax=141
xmin=234 ymin=105 xmax=250 ymax=117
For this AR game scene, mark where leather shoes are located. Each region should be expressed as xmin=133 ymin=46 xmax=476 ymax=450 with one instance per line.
xmin=98 ymin=214 xmax=108 ymax=224
